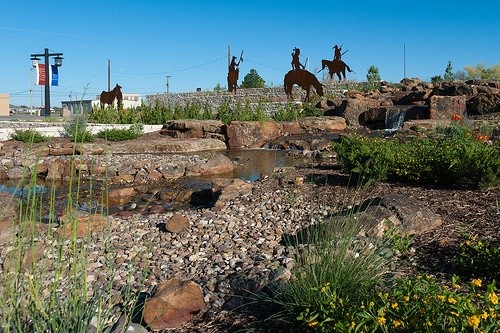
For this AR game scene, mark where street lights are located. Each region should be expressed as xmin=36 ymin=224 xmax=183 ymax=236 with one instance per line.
xmin=29 ymin=48 xmax=65 ymax=118
